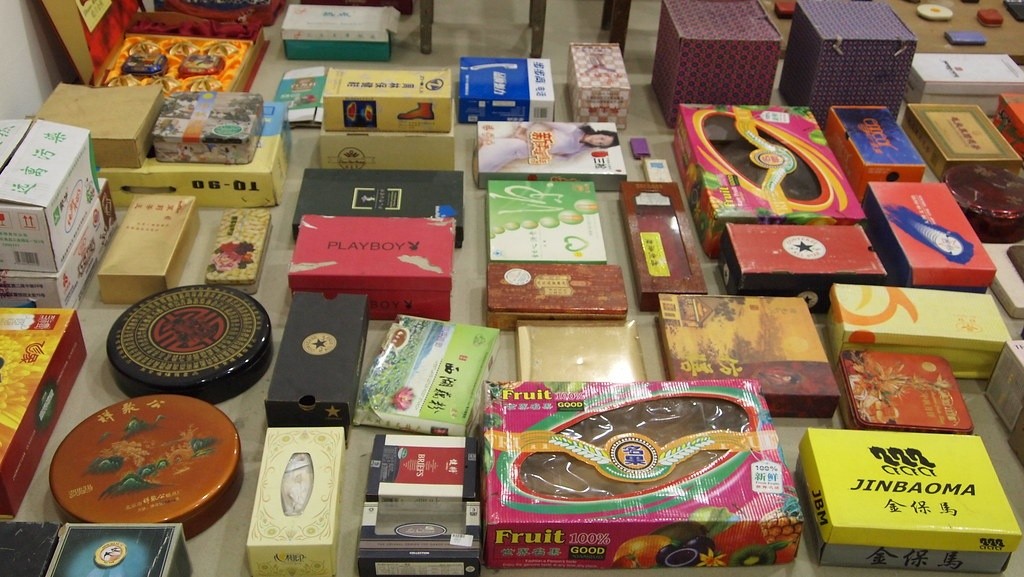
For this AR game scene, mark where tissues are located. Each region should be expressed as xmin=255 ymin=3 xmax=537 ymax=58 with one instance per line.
xmin=246 ymin=427 xmax=347 ymax=577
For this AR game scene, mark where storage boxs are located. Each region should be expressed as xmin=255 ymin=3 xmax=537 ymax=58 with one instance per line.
xmin=0 ymin=0 xmax=1024 ymax=577
